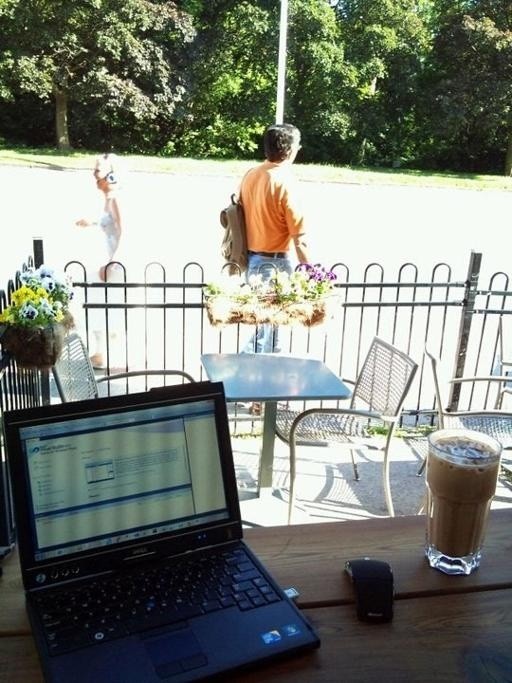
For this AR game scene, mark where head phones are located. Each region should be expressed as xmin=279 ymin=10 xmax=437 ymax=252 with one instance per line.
xmin=105 ymin=154 xmax=117 ymax=184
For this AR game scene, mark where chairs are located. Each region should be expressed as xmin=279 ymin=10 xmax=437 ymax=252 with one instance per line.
xmin=50 ymin=333 xmax=195 ymax=404
xmin=417 ymin=342 xmax=511 ymax=516
xmin=275 ymin=336 xmax=419 ymax=518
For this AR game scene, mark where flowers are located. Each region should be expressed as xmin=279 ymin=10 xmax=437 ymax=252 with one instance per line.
xmin=1 ymin=261 xmax=74 ymax=324
xmin=204 ymin=264 xmax=336 ymax=298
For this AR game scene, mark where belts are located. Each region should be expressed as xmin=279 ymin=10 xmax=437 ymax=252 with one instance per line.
xmin=248 ymin=250 xmax=287 ymax=258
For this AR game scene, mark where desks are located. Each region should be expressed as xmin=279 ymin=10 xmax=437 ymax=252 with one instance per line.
xmin=202 ymin=353 xmax=350 ymax=501
xmin=2 ymin=512 xmax=511 ymax=681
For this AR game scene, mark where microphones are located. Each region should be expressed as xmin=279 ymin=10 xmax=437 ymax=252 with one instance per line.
xmin=96 ymin=177 xmax=107 ymax=183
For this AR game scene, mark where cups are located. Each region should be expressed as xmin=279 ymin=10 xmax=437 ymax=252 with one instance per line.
xmin=423 ymin=430 xmax=502 ymax=576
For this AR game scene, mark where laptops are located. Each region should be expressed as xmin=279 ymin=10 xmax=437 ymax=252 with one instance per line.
xmin=2 ymin=381 xmax=320 ymax=682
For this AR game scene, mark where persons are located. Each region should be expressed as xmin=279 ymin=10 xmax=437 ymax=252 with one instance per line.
xmin=76 ymin=154 xmax=132 ymax=368
xmin=239 ymin=124 xmax=309 ymax=416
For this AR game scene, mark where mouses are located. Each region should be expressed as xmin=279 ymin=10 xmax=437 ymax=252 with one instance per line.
xmin=344 ymin=557 xmax=394 ymax=626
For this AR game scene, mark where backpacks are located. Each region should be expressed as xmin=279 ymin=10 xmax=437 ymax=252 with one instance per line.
xmin=220 ymin=167 xmax=253 ymax=276
